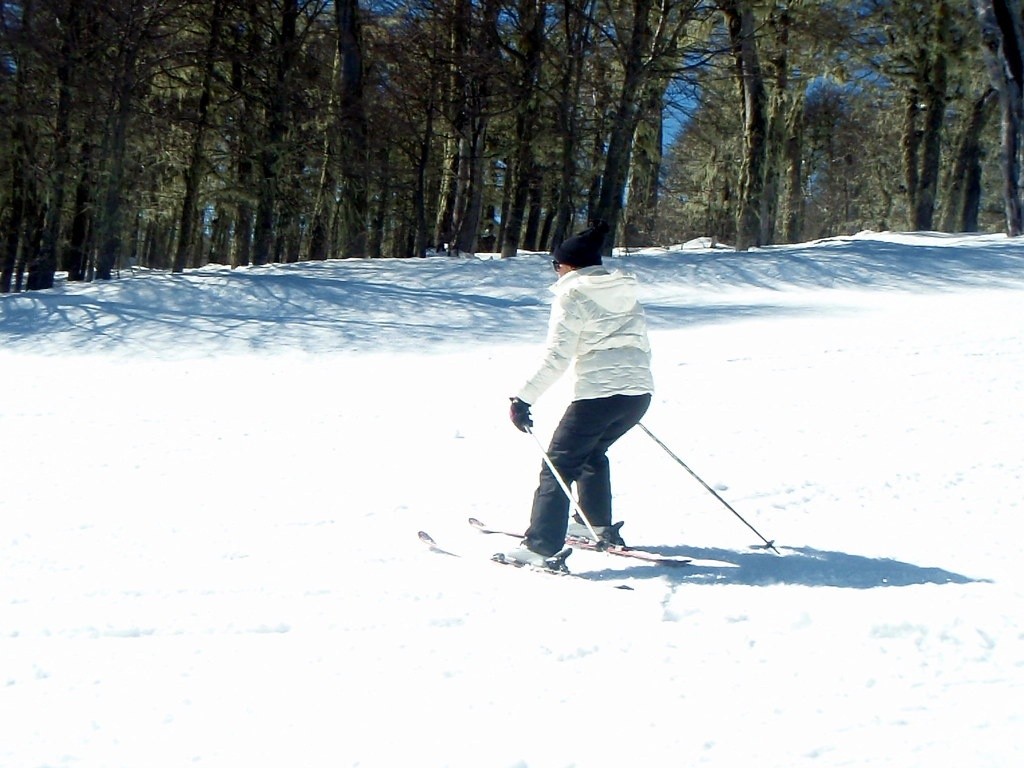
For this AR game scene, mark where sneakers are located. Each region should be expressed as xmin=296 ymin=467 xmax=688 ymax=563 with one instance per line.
xmin=567 ymin=514 xmax=607 ymax=539
xmin=509 ymin=543 xmax=549 ymax=566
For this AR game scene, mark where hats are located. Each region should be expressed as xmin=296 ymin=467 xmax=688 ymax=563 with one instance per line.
xmin=553 ymin=218 xmax=610 ymax=268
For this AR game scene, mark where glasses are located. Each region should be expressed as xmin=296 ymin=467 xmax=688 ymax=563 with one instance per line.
xmin=553 ymin=260 xmax=563 ymax=272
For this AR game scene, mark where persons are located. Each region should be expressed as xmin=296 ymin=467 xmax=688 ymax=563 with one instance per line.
xmin=505 ymin=220 xmax=654 ymax=568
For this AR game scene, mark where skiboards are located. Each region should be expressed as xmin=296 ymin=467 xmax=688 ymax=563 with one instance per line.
xmin=417 ymin=516 xmax=691 ymax=594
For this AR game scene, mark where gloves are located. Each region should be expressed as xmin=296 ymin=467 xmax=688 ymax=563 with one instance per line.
xmin=509 ymin=396 xmax=533 ymax=433
